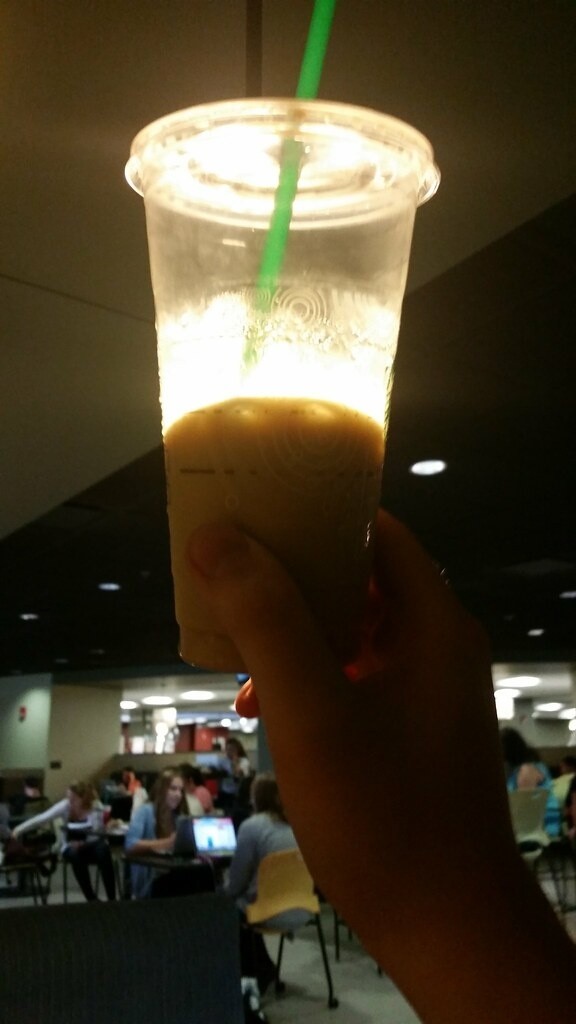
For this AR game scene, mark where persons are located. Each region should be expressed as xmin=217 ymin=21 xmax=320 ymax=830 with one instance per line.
xmin=498 ymin=727 xmax=576 ymax=843
xmin=0 ymin=736 xmax=317 ymax=992
xmin=188 ymin=511 xmax=576 ymax=1024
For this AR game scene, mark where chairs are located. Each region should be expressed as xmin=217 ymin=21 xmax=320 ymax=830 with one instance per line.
xmin=0 ymin=772 xmax=575 ymax=1009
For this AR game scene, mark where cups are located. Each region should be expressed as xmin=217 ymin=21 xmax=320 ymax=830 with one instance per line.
xmin=124 ymin=98 xmax=441 ymax=672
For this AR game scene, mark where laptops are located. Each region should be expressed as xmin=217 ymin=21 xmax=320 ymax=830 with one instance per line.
xmin=155 ymin=816 xmax=198 ymax=858
xmin=189 ymin=813 xmax=239 ymax=861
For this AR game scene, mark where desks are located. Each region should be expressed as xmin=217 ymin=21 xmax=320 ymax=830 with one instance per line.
xmin=124 ymin=852 xmax=236 ymax=870
xmin=62 ymin=820 xmax=130 ymax=840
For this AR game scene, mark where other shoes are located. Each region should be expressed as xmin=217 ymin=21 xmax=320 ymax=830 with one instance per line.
xmin=242 ymin=988 xmax=268 ymax=1024
